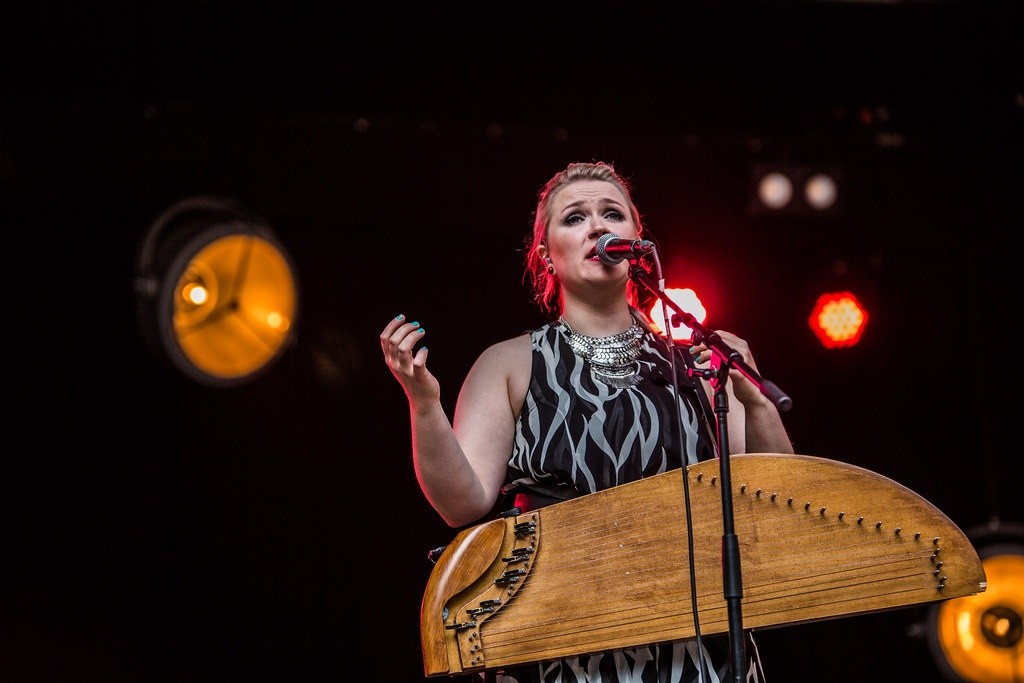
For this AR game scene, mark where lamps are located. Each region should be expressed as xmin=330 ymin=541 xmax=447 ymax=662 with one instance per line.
xmin=135 ymin=194 xmax=304 ymax=388
xmin=909 ymin=523 xmax=1024 ymax=683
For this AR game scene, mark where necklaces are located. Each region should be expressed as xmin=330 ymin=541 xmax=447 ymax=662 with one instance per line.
xmin=559 ymin=316 xmax=645 ymax=389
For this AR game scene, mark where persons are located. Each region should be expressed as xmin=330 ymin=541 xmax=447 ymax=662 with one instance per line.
xmin=379 ymin=161 xmax=795 ymax=683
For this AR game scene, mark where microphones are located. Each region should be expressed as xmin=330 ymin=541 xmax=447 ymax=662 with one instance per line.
xmin=596 ymin=232 xmax=656 ymax=265
